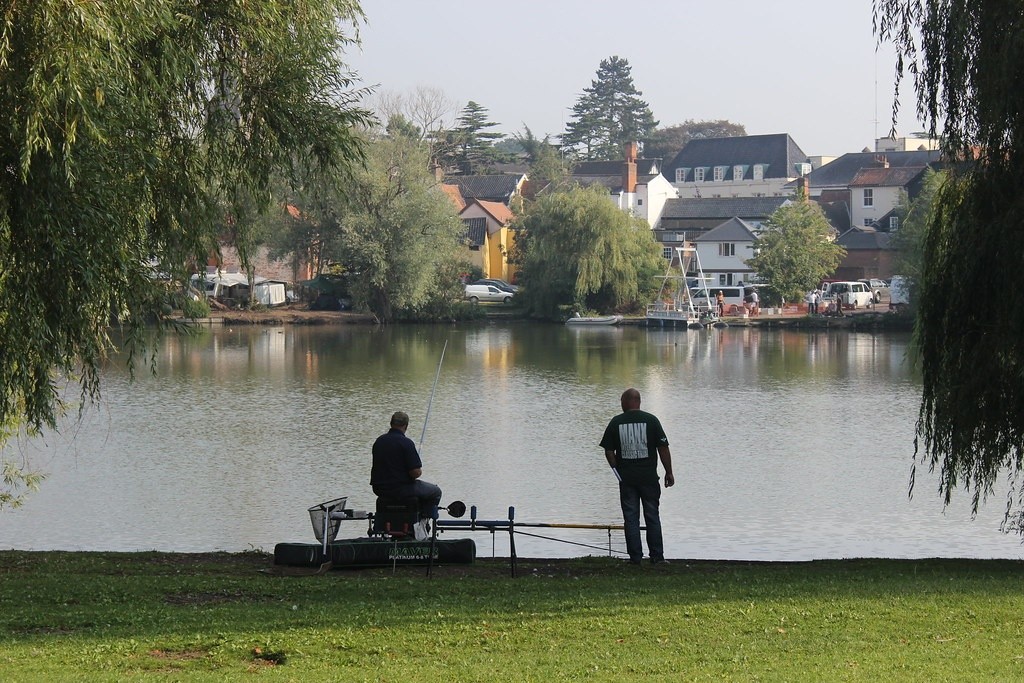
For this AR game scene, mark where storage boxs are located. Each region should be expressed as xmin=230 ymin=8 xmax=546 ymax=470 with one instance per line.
xmin=723 ymin=304 xmax=782 ymax=318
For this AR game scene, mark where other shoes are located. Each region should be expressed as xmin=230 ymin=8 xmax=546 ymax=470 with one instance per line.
xmin=653 ymin=559 xmax=671 ymax=568
xmin=630 ymin=557 xmax=643 ymax=565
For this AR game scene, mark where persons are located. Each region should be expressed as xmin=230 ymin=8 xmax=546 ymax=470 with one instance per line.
xmin=370 ymin=411 xmax=442 ymax=541
xmin=743 ymin=288 xmax=760 ymax=318
xmin=716 ymin=290 xmax=725 ymax=315
xmin=808 ymin=288 xmax=846 ymax=319
xmin=599 ymin=388 xmax=674 ymax=567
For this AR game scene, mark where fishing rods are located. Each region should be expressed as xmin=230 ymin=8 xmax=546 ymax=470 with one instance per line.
xmin=418 ymin=338 xmax=449 ymax=457
xmin=484 ymin=525 xmax=649 ymax=559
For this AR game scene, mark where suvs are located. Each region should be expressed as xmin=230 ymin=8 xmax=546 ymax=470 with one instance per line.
xmin=472 ymin=278 xmax=519 ymax=295
xmin=857 ymin=278 xmax=891 ymax=304
xmin=465 ymin=284 xmax=513 ymax=304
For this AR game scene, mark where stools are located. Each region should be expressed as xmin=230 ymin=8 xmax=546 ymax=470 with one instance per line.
xmin=374 ymin=498 xmax=419 ymax=536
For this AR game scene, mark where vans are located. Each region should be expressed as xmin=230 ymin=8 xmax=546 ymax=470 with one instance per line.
xmin=804 ymin=282 xmax=874 ymax=310
xmin=685 ymin=286 xmax=760 ymax=312
